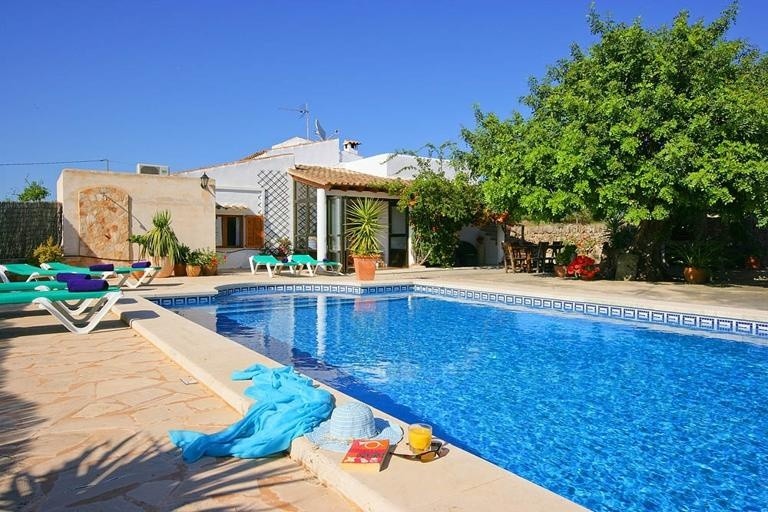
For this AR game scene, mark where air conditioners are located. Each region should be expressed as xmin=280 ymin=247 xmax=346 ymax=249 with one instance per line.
xmin=136 ymin=163 xmax=169 ymax=176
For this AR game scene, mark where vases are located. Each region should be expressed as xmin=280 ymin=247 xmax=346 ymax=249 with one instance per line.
xmin=275 ymin=256 xmax=285 ymax=261
xmin=202 ymin=264 xmax=216 ymax=275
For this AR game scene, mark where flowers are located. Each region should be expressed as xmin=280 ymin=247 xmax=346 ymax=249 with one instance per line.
xmin=259 ymin=237 xmax=292 ymax=257
xmin=201 ymin=250 xmax=227 ymax=265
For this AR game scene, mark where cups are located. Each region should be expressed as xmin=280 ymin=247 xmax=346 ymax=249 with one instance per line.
xmin=407 ymin=422 xmax=432 ymax=452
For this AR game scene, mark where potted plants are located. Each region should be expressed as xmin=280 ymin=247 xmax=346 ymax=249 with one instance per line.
xmin=186 ymin=252 xmax=201 ymax=276
xmin=343 ymin=196 xmax=389 ymax=280
xmin=142 ymin=210 xmax=180 ymax=278
xmin=741 ymin=241 xmax=764 ymax=270
xmin=668 ymin=240 xmax=726 ymax=284
xmin=173 ymin=244 xmax=190 ymax=276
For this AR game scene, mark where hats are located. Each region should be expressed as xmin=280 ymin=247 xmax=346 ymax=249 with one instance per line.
xmin=303 ymin=402 xmax=403 ymax=453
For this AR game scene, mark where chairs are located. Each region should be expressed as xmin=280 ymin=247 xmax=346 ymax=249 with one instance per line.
xmin=286 ymin=254 xmax=343 ymax=276
xmin=501 ymin=240 xmax=564 ymax=273
xmin=248 ymin=255 xmax=299 ymax=277
xmin=0 ymin=262 xmax=162 ymax=334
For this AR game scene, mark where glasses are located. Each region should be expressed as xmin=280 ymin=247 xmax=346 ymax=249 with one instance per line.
xmin=389 ymin=442 xmax=442 ymax=463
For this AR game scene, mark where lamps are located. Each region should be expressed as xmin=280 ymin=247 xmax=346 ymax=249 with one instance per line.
xmin=200 ymin=172 xmax=209 ymax=190
